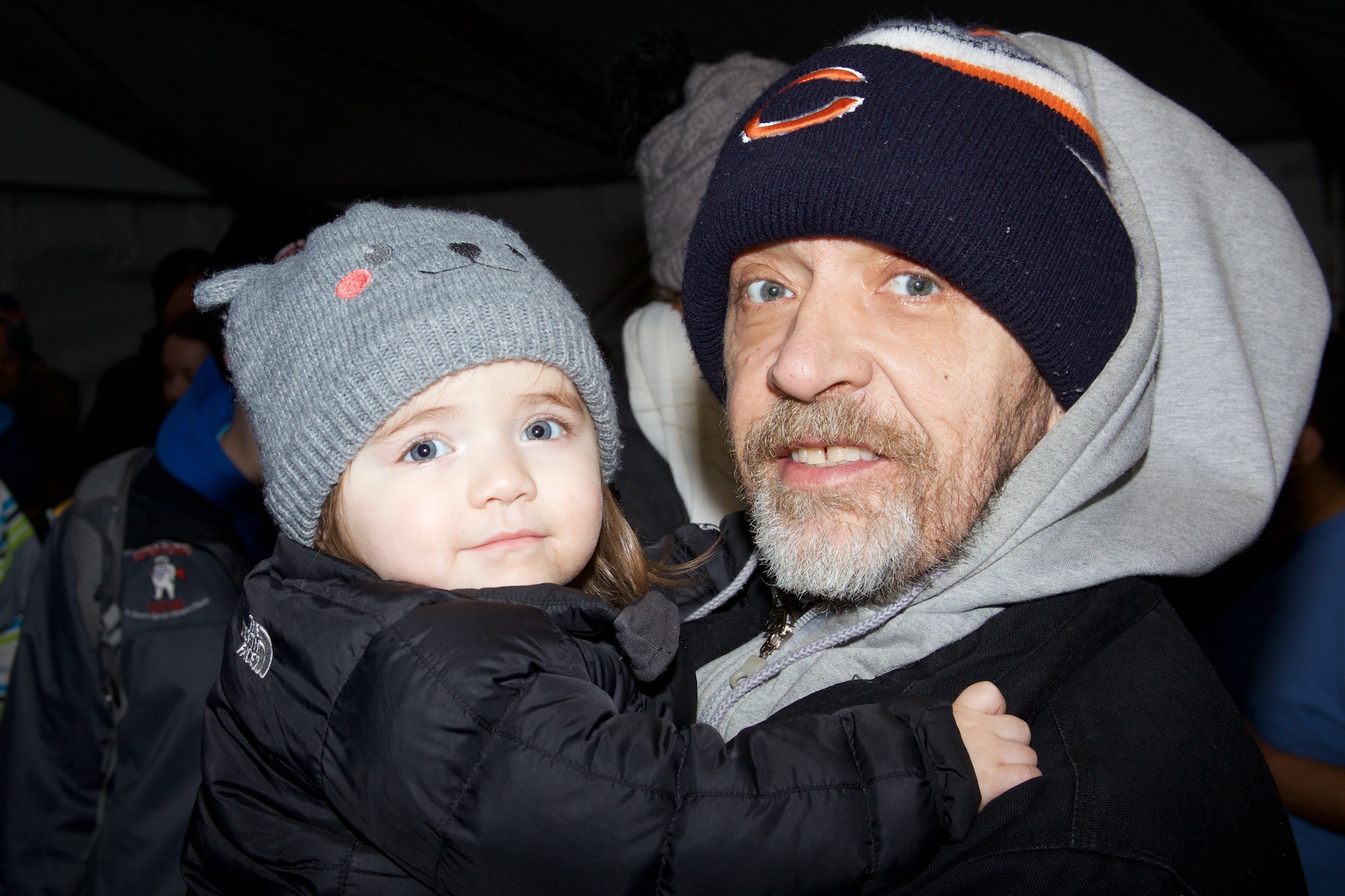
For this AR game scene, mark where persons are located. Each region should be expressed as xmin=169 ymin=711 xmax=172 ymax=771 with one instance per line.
xmin=1203 ymin=351 xmax=1345 ymax=896
xmin=174 ymin=201 xmax=1048 ymax=896
xmin=84 ymin=246 xmax=213 ymax=470
xmin=642 ymin=19 xmax=1338 ymax=896
xmin=0 ymin=403 xmax=49 ymax=715
xmin=157 ymin=320 xmax=213 ymax=398
xmin=603 ymin=55 xmax=800 ymax=552
xmin=2 ymin=202 xmax=334 ymax=896
xmin=0 ymin=292 xmax=84 ymax=438
xmin=2 ymin=318 xmax=93 ymax=548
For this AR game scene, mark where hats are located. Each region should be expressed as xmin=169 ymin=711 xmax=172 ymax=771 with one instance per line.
xmin=680 ymin=16 xmax=1140 ymax=412
xmin=189 ymin=200 xmax=619 ymax=549
xmin=636 ymin=55 xmax=791 ymax=292
